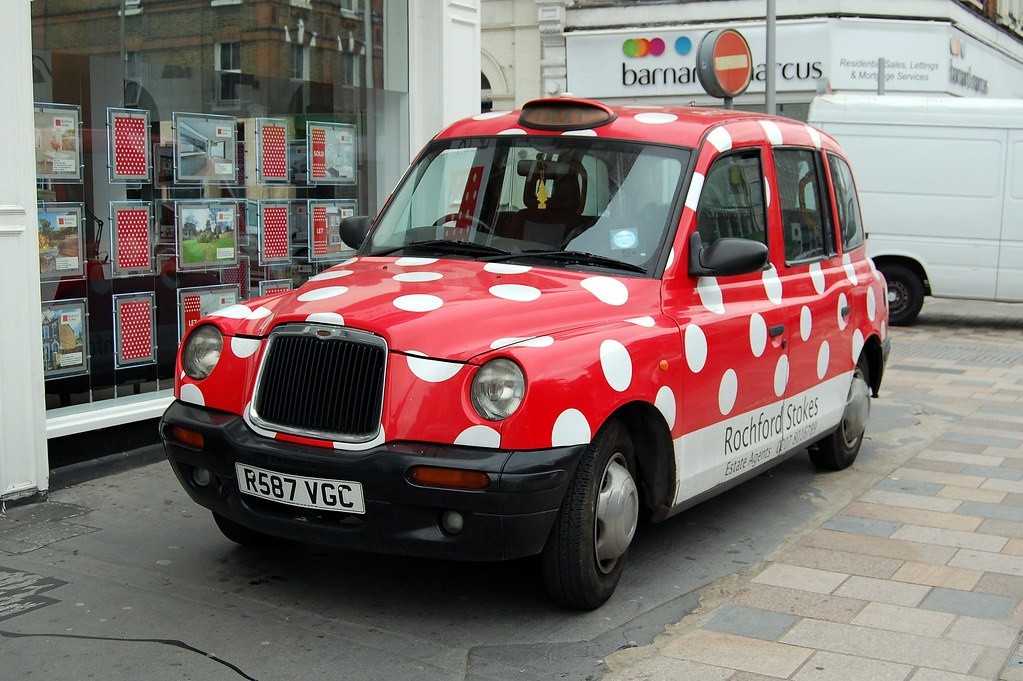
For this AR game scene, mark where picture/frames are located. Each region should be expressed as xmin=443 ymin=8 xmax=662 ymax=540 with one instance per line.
xmin=176 ymin=116 xmax=235 ymax=181
xmin=41 ymin=303 xmax=87 ymax=377
xmin=310 ymin=203 xmax=357 ymax=258
xmin=180 ymin=288 xmax=238 ymax=340
xmin=34 ymin=108 xmax=80 ymax=179
xmin=309 ymin=125 xmax=356 ymax=182
xmin=177 ymin=204 xmax=237 ymax=268
xmin=37 ymin=207 xmax=83 ymax=279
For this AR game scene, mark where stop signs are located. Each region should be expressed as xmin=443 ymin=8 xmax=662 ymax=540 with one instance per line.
xmin=696 ymin=29 xmax=755 ymax=97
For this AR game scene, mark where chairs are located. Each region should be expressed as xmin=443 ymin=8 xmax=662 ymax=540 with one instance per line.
xmin=499 ymin=159 xmax=588 ymax=253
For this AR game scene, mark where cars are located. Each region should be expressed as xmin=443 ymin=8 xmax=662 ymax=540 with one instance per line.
xmin=156 ymin=91 xmax=897 ymax=612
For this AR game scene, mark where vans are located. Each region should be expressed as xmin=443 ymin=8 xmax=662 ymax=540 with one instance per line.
xmin=806 ymin=92 xmax=1023 ymax=323
